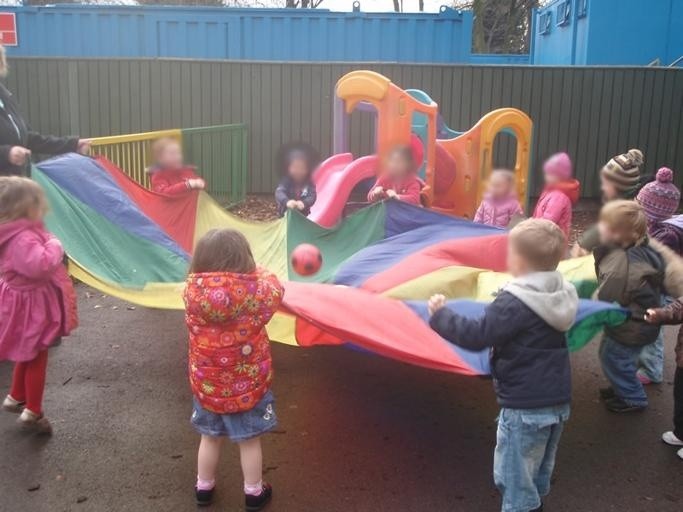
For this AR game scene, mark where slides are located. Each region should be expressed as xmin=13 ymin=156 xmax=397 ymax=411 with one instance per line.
xmin=305 ymin=152 xmax=380 ymax=229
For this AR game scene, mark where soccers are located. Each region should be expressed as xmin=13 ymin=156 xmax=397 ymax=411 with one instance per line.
xmin=290 ymin=243 xmax=322 ymax=276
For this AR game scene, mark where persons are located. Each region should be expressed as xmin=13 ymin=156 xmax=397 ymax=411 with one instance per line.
xmin=588 ymin=198 xmax=666 ymax=415
xmin=642 ymin=294 xmax=683 ymax=459
xmin=142 ymin=136 xmax=207 ymax=196
xmin=472 ymin=168 xmax=525 ymax=229
xmin=634 ymin=167 xmax=683 ymax=386
xmin=179 ymin=227 xmax=287 ymax=511
xmin=1 ymin=175 xmax=81 ymax=437
xmin=531 ymin=152 xmax=581 ymax=241
xmin=271 ymin=147 xmax=319 ymax=220
xmin=365 ymin=143 xmax=422 ymax=207
xmin=424 ymin=217 xmax=581 ymax=512
xmin=568 ymin=147 xmax=645 ymax=259
xmin=0 ymin=42 xmax=96 ymax=178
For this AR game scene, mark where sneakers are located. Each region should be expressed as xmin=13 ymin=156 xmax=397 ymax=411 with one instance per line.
xmin=195 ymin=485 xmax=215 ymax=505
xmin=661 ymin=431 xmax=683 ymax=445
xmin=598 ymin=386 xmax=643 ymax=412
xmin=2 ymin=394 xmax=26 ymax=414
xmin=15 ymin=408 xmax=52 ymax=433
xmin=245 ymin=483 xmax=272 ymax=511
xmin=636 ymin=371 xmax=651 ymax=385
xmin=676 ymin=447 xmax=683 ymax=459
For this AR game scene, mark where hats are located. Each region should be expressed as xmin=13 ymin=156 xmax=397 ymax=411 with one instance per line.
xmin=543 ymin=152 xmax=572 ymax=180
xmin=633 ymin=167 xmax=681 ymax=229
xmin=599 ymin=149 xmax=643 ymax=196
